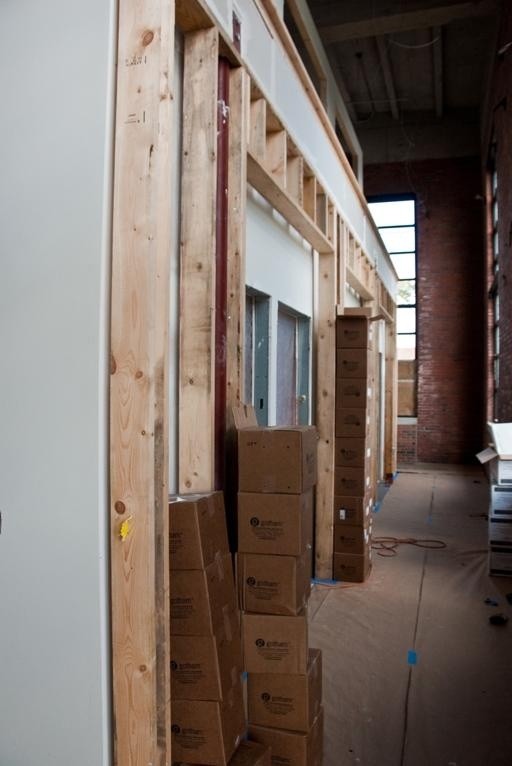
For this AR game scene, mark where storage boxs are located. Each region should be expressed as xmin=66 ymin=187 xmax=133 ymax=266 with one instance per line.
xmin=237 ymin=553 xmax=311 ymax=614
xmin=239 ymin=426 xmax=318 ymax=494
xmin=473 ymin=419 xmax=511 ymax=577
xmin=168 ymin=490 xmax=230 ymax=569
xmin=171 ymin=680 xmax=247 ymax=766
xmin=238 ymin=493 xmax=313 ymax=557
xmin=170 ymin=609 xmax=243 ymax=702
xmin=173 ymin=740 xmax=272 ymax=766
xmin=334 ymin=304 xmax=386 ymax=587
xmin=169 ymin=553 xmax=239 ymax=635
xmin=247 ymin=647 xmax=323 ymax=731
xmin=247 ymin=725 xmax=323 ymax=766
xmin=240 ymin=610 xmax=308 ymax=674
